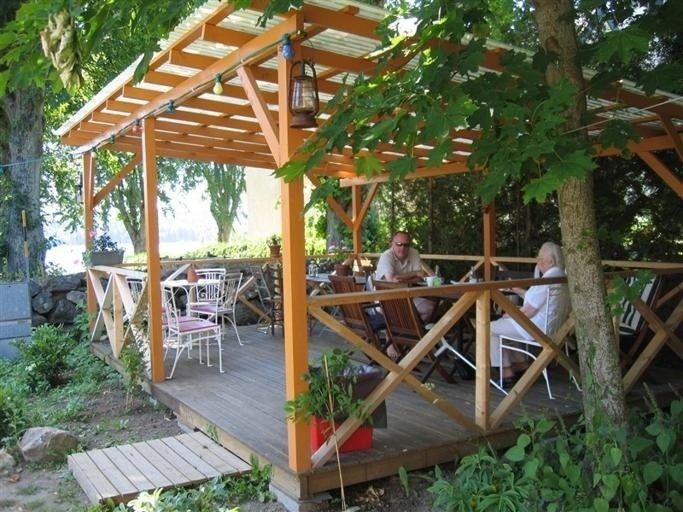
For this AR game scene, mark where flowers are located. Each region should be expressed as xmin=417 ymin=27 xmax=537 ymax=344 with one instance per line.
xmin=81 ymin=229 xmax=121 ymax=268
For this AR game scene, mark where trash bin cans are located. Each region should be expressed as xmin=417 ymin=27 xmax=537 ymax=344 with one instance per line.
xmin=308 ymin=359 xmax=381 ymax=452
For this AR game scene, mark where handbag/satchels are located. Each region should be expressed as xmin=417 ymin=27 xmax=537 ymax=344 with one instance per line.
xmin=454 ymin=352 xmax=475 ymax=379
xmin=365 ymin=312 xmax=386 ymax=331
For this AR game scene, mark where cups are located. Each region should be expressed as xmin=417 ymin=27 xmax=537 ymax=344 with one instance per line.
xmin=424 ymin=276 xmax=437 ymax=287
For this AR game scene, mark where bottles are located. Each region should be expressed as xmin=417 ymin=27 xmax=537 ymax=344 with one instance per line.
xmin=435 ymin=264 xmax=441 ymax=278
xmin=468 ymin=265 xmax=478 ymax=284
xmin=308 ymin=260 xmax=318 ymax=277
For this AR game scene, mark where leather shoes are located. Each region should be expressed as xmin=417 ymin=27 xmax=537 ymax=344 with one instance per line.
xmin=490 ymin=376 xmax=517 ymax=388
xmin=515 ymin=368 xmax=526 ymax=380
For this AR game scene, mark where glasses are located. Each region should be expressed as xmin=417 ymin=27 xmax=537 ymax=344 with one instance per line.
xmin=395 ymin=242 xmax=410 ymax=247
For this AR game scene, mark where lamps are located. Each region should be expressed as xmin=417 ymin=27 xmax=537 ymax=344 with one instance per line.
xmin=284 ymin=29 xmax=321 ymax=129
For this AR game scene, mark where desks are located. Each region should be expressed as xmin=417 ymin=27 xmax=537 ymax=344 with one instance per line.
xmin=374 ymin=278 xmax=518 ymax=376
xmin=159 ymin=279 xmax=221 ymax=350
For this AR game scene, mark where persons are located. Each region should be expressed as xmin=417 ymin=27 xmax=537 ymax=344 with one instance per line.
xmin=488 ymin=240 xmax=571 ymax=389
xmin=371 ymin=229 xmax=437 ymax=360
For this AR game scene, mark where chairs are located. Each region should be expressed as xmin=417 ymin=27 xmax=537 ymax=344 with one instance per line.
xmin=372 ymin=280 xmax=459 ymax=391
xmin=498 ymin=283 xmax=582 ymax=398
xmin=124 ymin=257 xmax=376 ymax=337
xmin=325 ymin=274 xmax=384 ymax=361
xmin=159 ymin=284 xmax=227 ymax=379
xmin=186 ymin=272 xmax=244 ymax=348
xmin=186 ymin=267 xmax=227 ymax=344
xmin=611 ymin=269 xmax=659 ymax=339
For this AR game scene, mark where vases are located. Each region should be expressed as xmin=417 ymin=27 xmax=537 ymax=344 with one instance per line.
xmin=82 ymin=250 xmax=125 ymax=266
